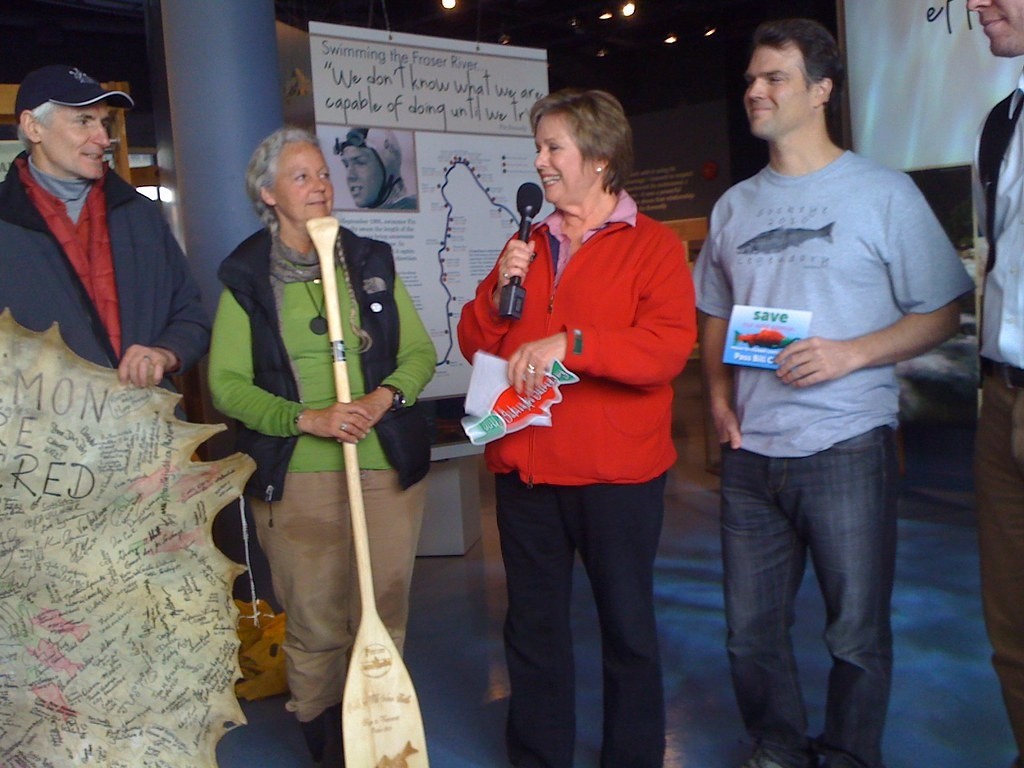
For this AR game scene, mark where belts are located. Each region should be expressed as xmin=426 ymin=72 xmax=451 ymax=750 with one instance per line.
xmin=981 ymin=357 xmax=1024 ymax=388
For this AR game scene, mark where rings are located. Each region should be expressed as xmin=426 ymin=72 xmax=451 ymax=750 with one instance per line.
xmin=503 ymin=273 xmax=509 ymax=279
xmin=144 ymin=355 xmax=151 ymax=362
xmin=526 ymin=363 xmax=535 ymax=374
xmin=340 ymin=423 xmax=347 ymax=430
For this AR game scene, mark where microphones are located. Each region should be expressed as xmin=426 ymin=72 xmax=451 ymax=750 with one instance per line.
xmin=498 ymin=183 xmax=543 ymax=319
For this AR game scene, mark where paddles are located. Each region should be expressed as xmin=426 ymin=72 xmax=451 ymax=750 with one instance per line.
xmin=305 ymin=216 xmax=430 ymax=768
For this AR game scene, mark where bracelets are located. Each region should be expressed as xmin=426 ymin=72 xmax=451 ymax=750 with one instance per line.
xmin=294 ymin=404 xmax=304 ymax=434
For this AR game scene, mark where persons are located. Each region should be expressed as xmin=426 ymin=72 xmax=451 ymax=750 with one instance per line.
xmin=691 ymin=19 xmax=975 ymax=768
xmin=457 ymin=89 xmax=697 ymax=768
xmin=0 ymin=66 xmax=212 ymax=465
xmin=966 ymin=0 xmax=1024 ymax=768
xmin=208 ymin=128 xmax=437 ymax=768
xmin=331 ymin=127 xmax=417 ymax=210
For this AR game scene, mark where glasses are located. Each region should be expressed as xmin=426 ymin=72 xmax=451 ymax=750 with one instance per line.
xmin=334 ymin=131 xmax=365 ymax=155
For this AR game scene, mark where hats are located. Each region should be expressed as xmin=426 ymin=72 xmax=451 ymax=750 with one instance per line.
xmin=14 ymin=65 xmax=134 ymax=122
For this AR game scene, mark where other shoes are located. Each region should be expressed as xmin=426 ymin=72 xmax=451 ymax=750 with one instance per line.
xmin=739 ymin=756 xmax=783 ymax=768
xmin=303 ymin=703 xmax=345 ymax=768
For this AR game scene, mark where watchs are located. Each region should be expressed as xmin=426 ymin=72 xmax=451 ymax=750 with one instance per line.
xmin=379 ymin=383 xmax=405 ymax=411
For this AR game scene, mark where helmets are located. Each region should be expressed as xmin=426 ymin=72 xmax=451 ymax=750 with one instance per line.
xmin=335 ymin=128 xmax=403 ymax=174
